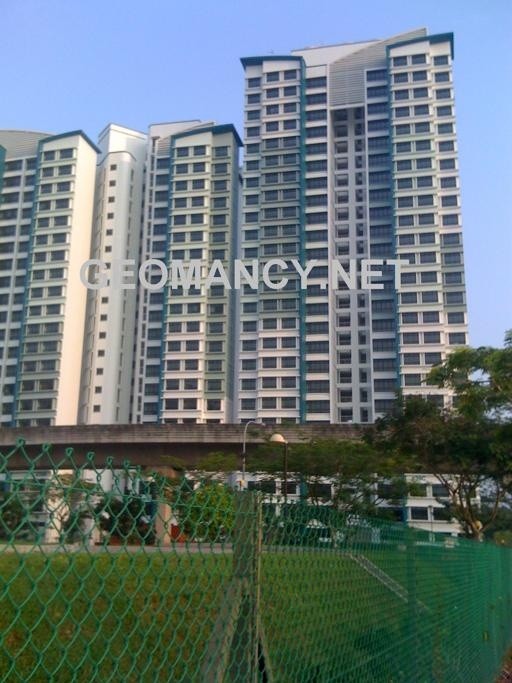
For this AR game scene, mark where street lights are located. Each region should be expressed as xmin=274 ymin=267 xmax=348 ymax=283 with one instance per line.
xmin=270 ymin=433 xmax=290 ymax=521
xmin=241 ymin=421 xmax=265 ymax=487
